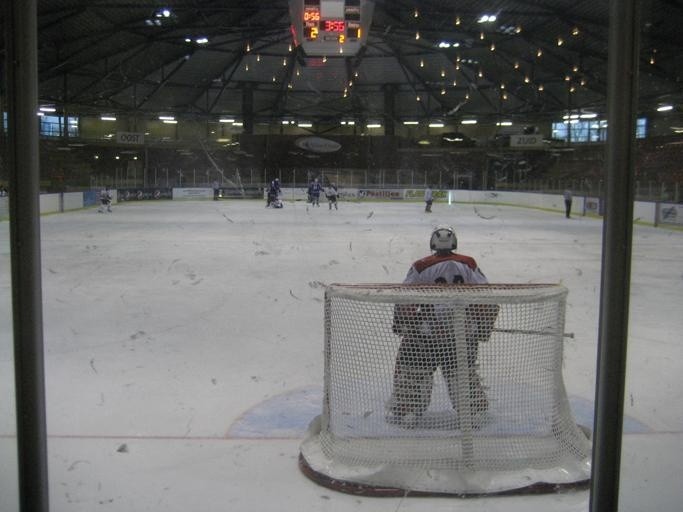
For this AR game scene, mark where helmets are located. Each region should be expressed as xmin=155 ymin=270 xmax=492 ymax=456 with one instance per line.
xmin=430 ymin=225 xmax=457 ymax=251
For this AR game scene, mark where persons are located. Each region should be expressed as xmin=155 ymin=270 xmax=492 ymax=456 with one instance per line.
xmin=423 ymin=185 xmax=433 ymax=214
xmin=269 ymin=178 xmax=281 ymax=201
xmin=563 ymin=188 xmax=574 ymax=219
xmin=324 ymin=183 xmax=338 ymax=210
xmin=306 ymin=178 xmax=323 ymax=207
xmin=331 ymin=182 xmax=339 ymax=198
xmin=212 ymin=177 xmax=221 ymax=200
xmin=265 ymin=186 xmax=283 ymax=208
xmin=97 ymin=188 xmax=112 ymax=213
xmin=384 ymin=228 xmax=500 ymax=417
